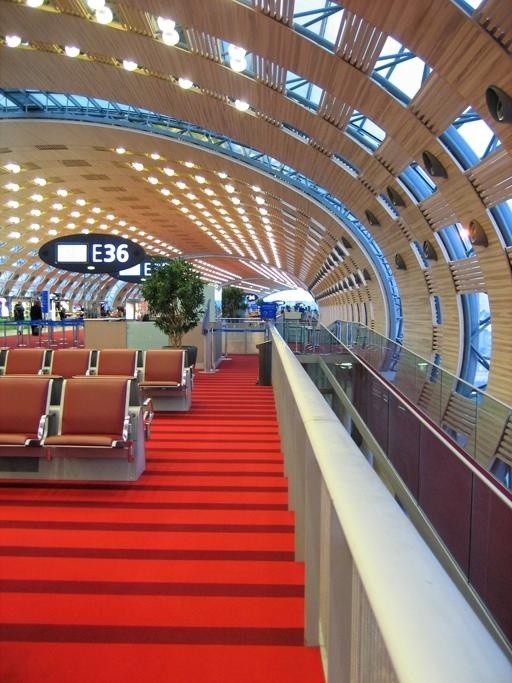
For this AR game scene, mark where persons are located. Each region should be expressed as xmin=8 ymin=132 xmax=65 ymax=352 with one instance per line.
xmin=14 ymin=299 xmax=25 ymax=334
xmin=30 ymin=300 xmax=42 ymax=333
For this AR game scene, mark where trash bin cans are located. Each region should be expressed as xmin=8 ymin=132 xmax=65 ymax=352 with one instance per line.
xmin=256 ymin=340 xmax=272 ymax=386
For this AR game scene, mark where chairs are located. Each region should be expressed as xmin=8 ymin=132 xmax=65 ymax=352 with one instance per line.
xmin=0 ymin=346 xmax=193 ymax=462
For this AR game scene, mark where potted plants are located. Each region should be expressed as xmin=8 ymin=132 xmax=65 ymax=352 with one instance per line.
xmin=144 ymin=254 xmax=206 ymax=367
